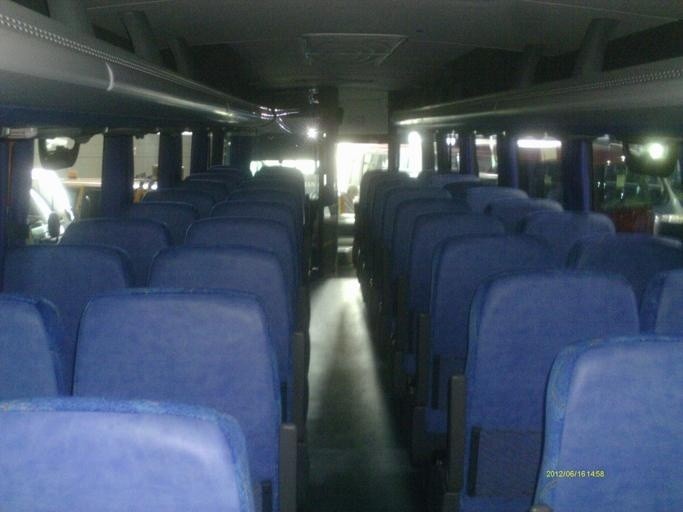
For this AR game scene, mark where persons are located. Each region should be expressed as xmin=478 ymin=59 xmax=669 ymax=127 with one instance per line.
xmin=340 ymin=186 xmax=358 ymax=213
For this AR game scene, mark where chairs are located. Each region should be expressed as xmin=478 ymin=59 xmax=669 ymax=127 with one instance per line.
xmin=442 ymin=265 xmax=643 ymax=512
xmin=140 ymin=242 xmax=315 ymax=469
xmin=5 ymin=238 xmax=142 ymax=342
xmin=1 ymin=393 xmax=261 ymax=512
xmin=523 ymin=330 xmax=682 ymax=511
xmin=0 ymin=287 xmax=74 ymax=398
xmin=410 ymin=230 xmax=552 ymax=473
xmin=72 ymin=282 xmax=304 ymax=512
xmin=639 ymin=269 xmax=681 ymax=336
xmin=56 ymin=153 xmax=316 ymax=365
xmin=349 ymin=163 xmax=621 ymax=420
xmin=566 ymin=231 xmax=671 ymax=292
